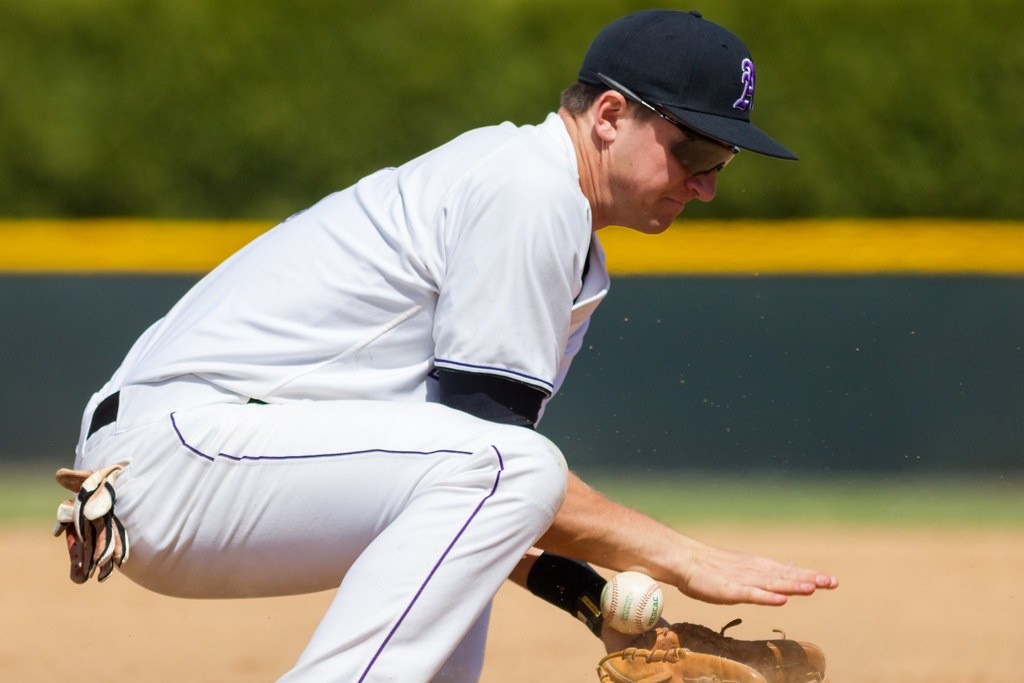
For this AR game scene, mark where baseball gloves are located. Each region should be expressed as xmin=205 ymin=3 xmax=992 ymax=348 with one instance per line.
xmin=594 ymin=620 xmax=827 ymax=683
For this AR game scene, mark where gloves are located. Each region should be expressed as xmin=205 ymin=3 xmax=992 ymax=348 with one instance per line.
xmin=51 ymin=451 xmax=129 ymax=580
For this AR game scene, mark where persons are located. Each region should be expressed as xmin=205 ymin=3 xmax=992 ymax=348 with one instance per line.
xmin=76 ymin=9 xmax=840 ymax=683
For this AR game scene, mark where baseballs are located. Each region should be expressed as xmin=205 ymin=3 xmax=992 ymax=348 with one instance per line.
xmin=599 ymin=570 xmax=665 ymax=636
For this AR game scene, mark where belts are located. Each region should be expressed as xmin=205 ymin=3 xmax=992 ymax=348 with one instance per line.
xmin=86 ymin=394 xmax=124 ymax=434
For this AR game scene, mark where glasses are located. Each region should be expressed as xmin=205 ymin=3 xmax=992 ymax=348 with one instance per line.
xmin=594 ymin=64 xmax=740 ymax=178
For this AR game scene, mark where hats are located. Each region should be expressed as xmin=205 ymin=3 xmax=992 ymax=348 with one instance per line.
xmin=574 ymin=9 xmax=800 ymax=162
